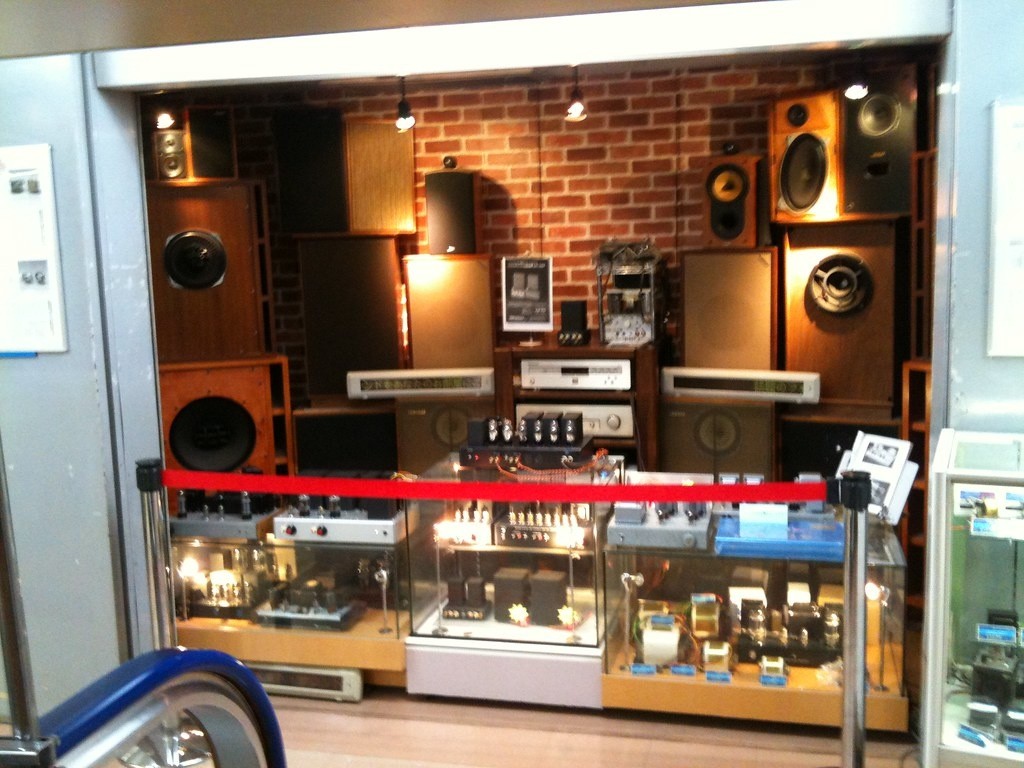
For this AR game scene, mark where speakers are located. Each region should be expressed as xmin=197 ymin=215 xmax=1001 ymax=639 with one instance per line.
xmin=182 ymin=105 xmax=238 ymax=182
xmin=145 ymin=181 xmax=267 ymax=365
xmin=468 ymin=577 xmax=485 ymax=607
xmin=840 ymin=62 xmax=928 ymax=214
xmin=659 ymin=397 xmax=776 ymax=483
xmin=298 ymin=238 xmax=402 ymax=399
xmin=158 ymin=362 xmax=277 ymax=517
xmin=767 ymin=89 xmax=842 ymax=222
xmin=530 ymin=570 xmax=567 ymax=625
xmin=448 ymin=576 xmax=465 ymax=606
xmin=401 ymin=253 xmax=497 ymax=369
xmin=701 ymin=155 xmax=765 ymax=249
xmin=679 ymin=246 xmax=777 ymax=370
xmin=152 ymin=131 xmax=188 ymax=180
xmin=494 ymin=567 xmax=529 ymax=622
xmin=425 ymin=169 xmax=483 ymax=254
xmin=783 ymin=223 xmax=896 ymax=405
xmin=278 ymin=106 xmax=349 ymax=234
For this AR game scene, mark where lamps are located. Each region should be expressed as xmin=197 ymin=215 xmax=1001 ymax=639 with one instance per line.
xmin=396 ymin=77 xmax=415 ymax=130
xmin=562 ymin=65 xmax=587 ymax=123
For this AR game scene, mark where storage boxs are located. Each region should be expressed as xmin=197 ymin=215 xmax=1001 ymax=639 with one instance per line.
xmin=714 ymin=517 xmax=844 ymax=562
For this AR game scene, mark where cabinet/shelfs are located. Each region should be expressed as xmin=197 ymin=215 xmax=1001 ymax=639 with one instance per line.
xmin=494 ymin=327 xmax=669 ymax=472
xmin=405 ymin=452 xmax=636 ymax=710
